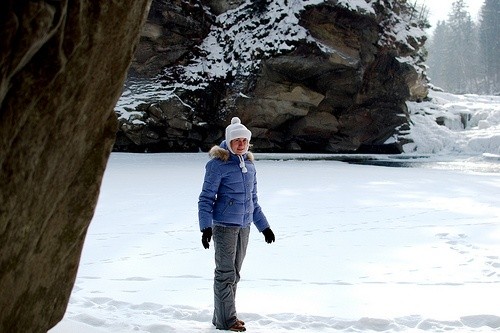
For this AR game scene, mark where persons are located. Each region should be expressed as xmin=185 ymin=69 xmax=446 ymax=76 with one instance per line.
xmin=198 ymin=116 xmax=275 ymax=332
